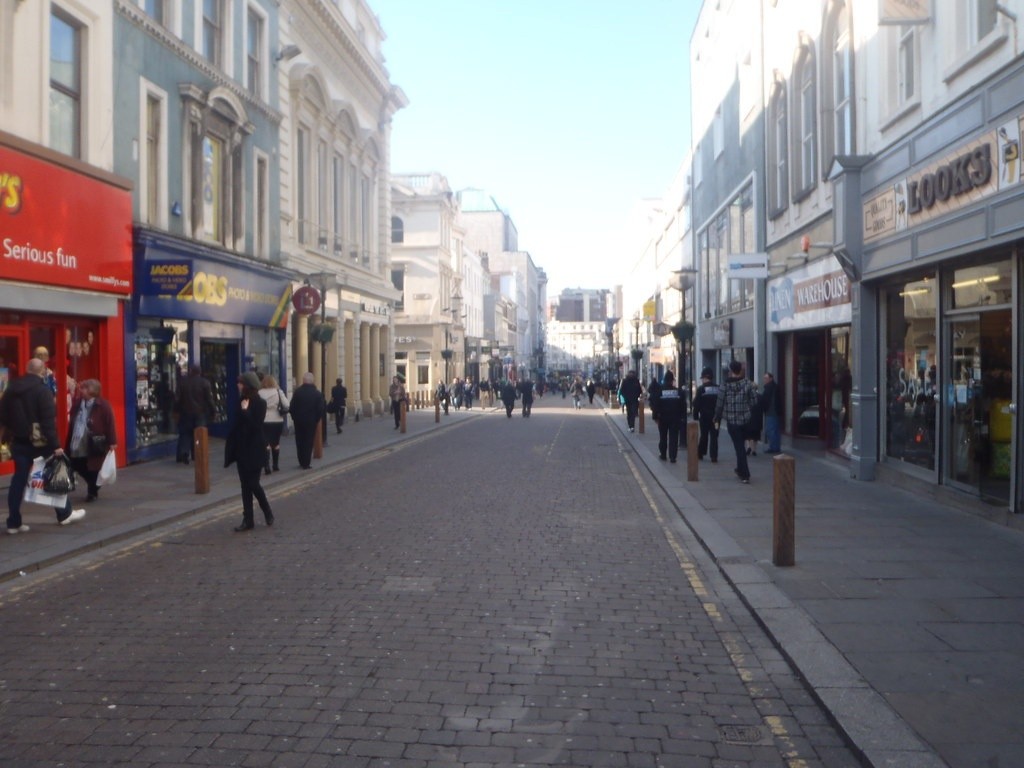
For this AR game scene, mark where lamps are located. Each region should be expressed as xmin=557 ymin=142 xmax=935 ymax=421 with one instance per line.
xmin=171 ymin=201 xmax=182 ymax=215
xmin=273 ymin=44 xmax=300 ymax=65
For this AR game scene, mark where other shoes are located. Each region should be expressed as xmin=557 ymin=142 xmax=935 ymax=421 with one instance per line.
xmin=741 ymin=478 xmax=748 ymax=483
xmin=764 ymin=447 xmax=780 ymax=453
xmin=659 ymin=451 xmax=717 ymax=464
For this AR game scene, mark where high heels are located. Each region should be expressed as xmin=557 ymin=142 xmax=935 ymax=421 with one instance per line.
xmin=752 ymin=451 xmax=757 ymax=456
xmin=83 ymin=493 xmax=98 ymax=502
xmin=746 ymin=447 xmax=751 ymax=457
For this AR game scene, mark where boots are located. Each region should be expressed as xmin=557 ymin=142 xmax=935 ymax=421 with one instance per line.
xmin=236 ymin=511 xmax=254 ymax=531
xmin=263 ymin=449 xmax=271 ymax=473
xmin=260 ymin=503 xmax=274 ymax=525
xmin=271 ymin=449 xmax=280 ymax=471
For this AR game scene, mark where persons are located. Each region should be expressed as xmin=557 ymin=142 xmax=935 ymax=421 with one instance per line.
xmin=587 ymin=382 xmax=596 ymax=404
xmin=569 ymin=376 xmax=585 ymax=409
xmin=762 ymin=372 xmax=782 ymax=453
xmin=620 ymin=370 xmax=642 ymax=433
xmin=258 ymin=376 xmax=290 ymax=476
xmin=436 ymin=376 xmax=474 ymax=411
xmin=539 ymin=381 xmax=557 ymax=398
xmin=561 ymin=378 xmax=569 ymax=399
xmin=693 ymin=368 xmax=720 ymax=462
xmin=225 ymin=373 xmax=274 ymax=531
xmin=648 ymin=378 xmax=660 ymax=411
xmin=332 ymin=378 xmax=348 ymax=434
xmin=289 ymin=372 xmax=326 ymax=469
xmin=389 ymin=376 xmax=407 ymax=429
xmin=713 ymin=362 xmax=758 ymax=484
xmin=617 ymin=379 xmax=626 ymax=413
xmin=0 ymin=358 xmax=86 ymax=533
xmin=652 ymin=370 xmax=685 ymax=463
xmin=180 ymin=365 xmax=217 ymax=466
xmin=501 ymin=379 xmax=537 ymax=417
xmin=64 ymin=378 xmax=118 ymax=501
xmin=480 ymin=376 xmax=488 ymax=392
xmin=35 ymin=346 xmax=57 ymax=395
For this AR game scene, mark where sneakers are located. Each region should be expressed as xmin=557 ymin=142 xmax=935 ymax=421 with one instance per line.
xmin=7 ymin=524 xmax=29 ymax=533
xmin=58 ymin=509 xmax=85 ymax=525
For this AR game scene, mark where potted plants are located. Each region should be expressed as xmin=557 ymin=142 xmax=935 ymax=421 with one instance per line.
xmin=441 ymin=350 xmax=452 ymax=359
xmin=311 ymin=323 xmax=334 ymax=341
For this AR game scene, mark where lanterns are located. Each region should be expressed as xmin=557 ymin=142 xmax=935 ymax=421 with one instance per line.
xmin=293 ymin=287 xmax=320 ymax=317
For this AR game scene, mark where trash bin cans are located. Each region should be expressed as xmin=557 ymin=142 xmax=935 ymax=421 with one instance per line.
xmin=610 ymin=390 xmax=619 ymax=409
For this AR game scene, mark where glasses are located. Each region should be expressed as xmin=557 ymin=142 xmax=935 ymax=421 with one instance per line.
xmin=700 ymin=376 xmax=706 ymax=380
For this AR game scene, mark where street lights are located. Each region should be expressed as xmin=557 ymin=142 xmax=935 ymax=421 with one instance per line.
xmin=613 ymin=339 xmax=624 ymax=389
xmin=439 ymin=320 xmax=456 ymax=392
xmin=310 ymin=267 xmax=337 ymax=444
xmin=631 ymin=311 xmax=644 ymax=378
xmin=668 ymin=265 xmax=698 ymax=414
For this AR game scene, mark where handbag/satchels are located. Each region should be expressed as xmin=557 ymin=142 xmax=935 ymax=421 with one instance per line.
xmin=23 ymin=457 xmax=68 ymax=508
xmin=31 ymin=422 xmax=49 ymax=448
xmin=92 ymin=435 xmax=109 ymax=456
xmin=326 ymin=402 xmax=341 ymax=414
xmin=94 ymin=447 xmax=116 ymax=486
xmin=277 ymin=388 xmax=290 ymax=416
xmin=44 ymin=451 xmax=75 ymax=494
